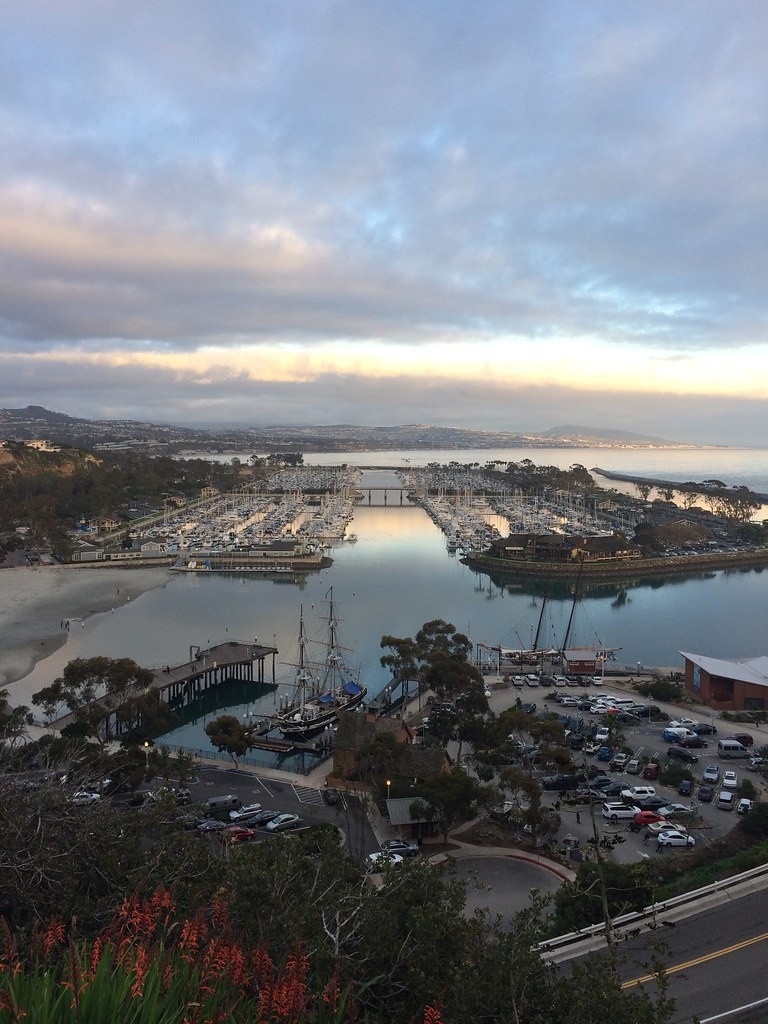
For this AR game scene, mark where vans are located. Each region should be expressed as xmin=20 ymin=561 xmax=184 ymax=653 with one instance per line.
xmin=203 ymin=794 xmax=242 ymax=819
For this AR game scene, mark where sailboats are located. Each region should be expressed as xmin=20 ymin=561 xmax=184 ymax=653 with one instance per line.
xmin=476 ymin=553 xmax=624 ymax=664
xmin=242 ymin=584 xmax=368 ymax=740
xmin=125 ymin=458 xmax=363 ymax=576
xmin=393 ymin=457 xmax=768 ymax=564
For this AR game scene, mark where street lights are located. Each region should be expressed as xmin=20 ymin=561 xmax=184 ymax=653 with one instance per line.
xmin=145 ymin=741 xmax=150 ymax=774
xmin=387 ymin=779 xmax=391 ymax=799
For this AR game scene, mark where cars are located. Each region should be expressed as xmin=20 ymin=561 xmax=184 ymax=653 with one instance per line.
xmin=229 ymin=802 xmax=264 ymax=823
xmin=561 ymin=837 xmax=580 ymax=854
xmin=656 ymin=831 xmax=696 ymax=846
xmin=410 ymin=669 xmax=768 ymax=831
xmin=325 ymin=788 xmax=339 ymax=805
xmin=13 ymin=768 xmax=112 ymax=807
xmin=174 ymin=814 xmax=204 ymax=829
xmin=216 ymin=825 xmax=256 ymax=845
xmin=266 ymin=813 xmax=301 ymax=833
xmin=246 ymin=808 xmax=282 ymax=828
xmin=197 ymin=819 xmax=229 ymax=836
xmin=144 ymin=785 xmax=193 ymax=808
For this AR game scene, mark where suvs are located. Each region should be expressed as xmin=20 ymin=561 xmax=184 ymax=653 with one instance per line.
xmin=365 ymin=853 xmax=404 ymax=874
xmin=381 ymin=839 xmax=420 ymax=857
